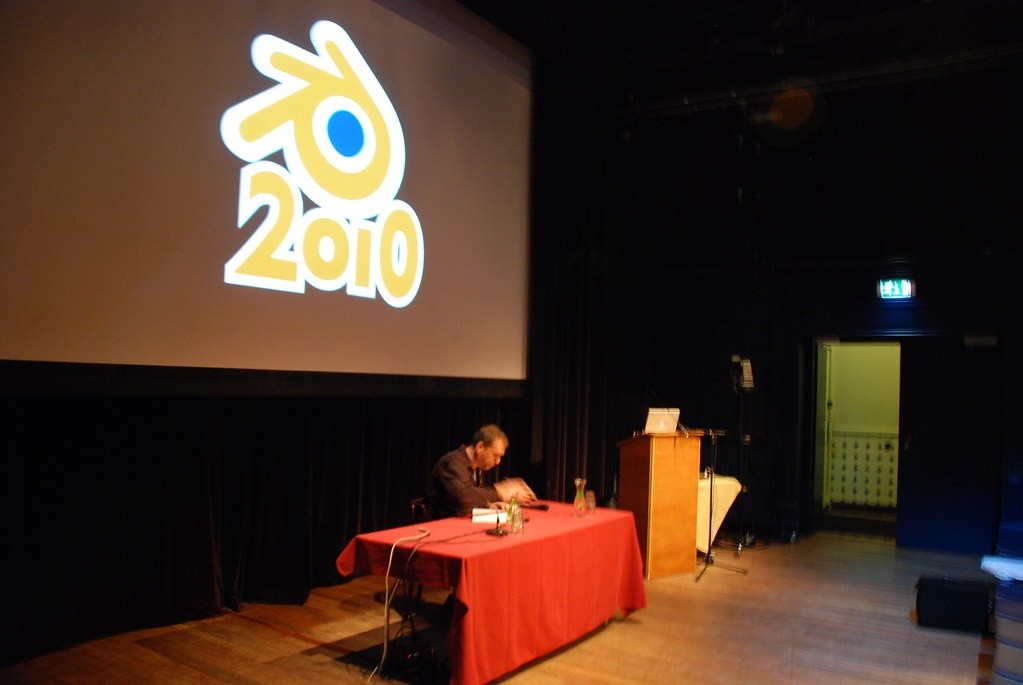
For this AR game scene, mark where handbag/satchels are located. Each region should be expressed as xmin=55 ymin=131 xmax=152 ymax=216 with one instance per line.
xmin=913 ymin=574 xmax=991 ymax=634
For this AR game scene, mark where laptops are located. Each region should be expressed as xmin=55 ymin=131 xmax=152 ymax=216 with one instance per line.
xmin=631 ymin=406 xmax=681 ymax=436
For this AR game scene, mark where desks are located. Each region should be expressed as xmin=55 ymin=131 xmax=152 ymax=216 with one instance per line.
xmin=335 ymin=500 xmax=648 ymax=685
xmin=696 ymin=472 xmax=742 ymax=560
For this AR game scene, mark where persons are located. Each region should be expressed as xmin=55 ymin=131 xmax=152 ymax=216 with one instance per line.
xmin=424 ymin=423 xmax=536 ymax=518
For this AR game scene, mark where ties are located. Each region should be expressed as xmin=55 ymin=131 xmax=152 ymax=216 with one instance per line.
xmin=475 ymin=468 xmax=480 ymax=487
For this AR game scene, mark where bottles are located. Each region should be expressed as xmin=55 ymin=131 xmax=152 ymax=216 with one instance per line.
xmin=507 ymin=489 xmax=518 ymax=525
xmin=575 ymin=478 xmax=587 ymax=516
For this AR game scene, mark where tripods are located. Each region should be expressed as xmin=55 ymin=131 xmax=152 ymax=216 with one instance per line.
xmin=695 ymin=433 xmax=750 ymax=582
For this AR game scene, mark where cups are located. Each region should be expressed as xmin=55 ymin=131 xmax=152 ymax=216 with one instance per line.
xmin=584 ymin=490 xmax=596 ymax=516
xmin=511 ymin=508 xmax=523 ymax=533
